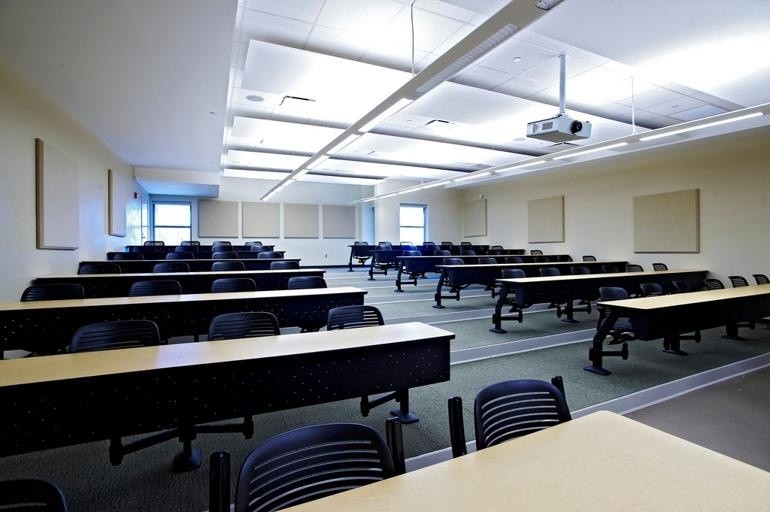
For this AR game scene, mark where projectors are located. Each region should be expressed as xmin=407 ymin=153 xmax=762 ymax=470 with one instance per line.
xmin=527 ymin=115 xmax=591 ymax=142
